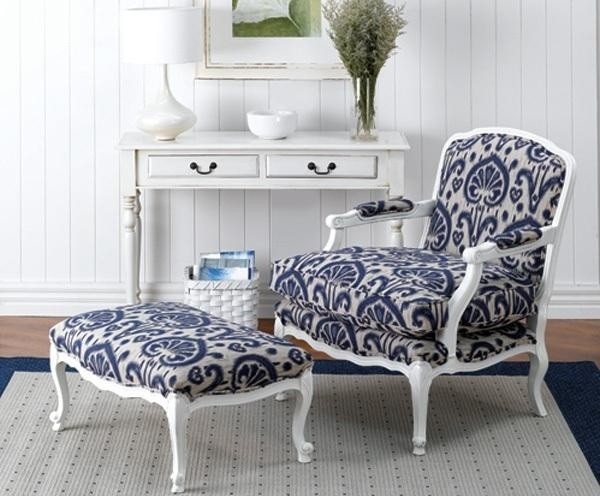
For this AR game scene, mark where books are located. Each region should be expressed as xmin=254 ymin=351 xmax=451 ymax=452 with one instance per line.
xmin=193 ymin=250 xmax=255 ymax=280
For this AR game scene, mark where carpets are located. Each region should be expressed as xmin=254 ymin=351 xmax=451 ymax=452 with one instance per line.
xmin=1 ymin=355 xmax=600 ymax=495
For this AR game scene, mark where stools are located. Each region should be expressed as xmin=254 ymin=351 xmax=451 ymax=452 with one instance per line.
xmin=47 ymin=302 xmax=315 ymax=494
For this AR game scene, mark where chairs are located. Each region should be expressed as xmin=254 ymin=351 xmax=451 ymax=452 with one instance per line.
xmin=269 ymin=125 xmax=578 ymax=458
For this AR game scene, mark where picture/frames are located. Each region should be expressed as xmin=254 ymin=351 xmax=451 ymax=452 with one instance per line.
xmin=191 ymin=0 xmax=353 ymax=81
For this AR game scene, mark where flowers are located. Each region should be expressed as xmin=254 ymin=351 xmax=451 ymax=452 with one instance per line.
xmin=320 ymin=0 xmax=409 ymax=133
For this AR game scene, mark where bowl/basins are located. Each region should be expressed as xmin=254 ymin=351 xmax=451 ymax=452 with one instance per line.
xmin=246 ymin=109 xmax=297 ymax=141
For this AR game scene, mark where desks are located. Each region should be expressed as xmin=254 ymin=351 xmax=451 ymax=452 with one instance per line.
xmin=121 ymin=132 xmax=411 ymax=306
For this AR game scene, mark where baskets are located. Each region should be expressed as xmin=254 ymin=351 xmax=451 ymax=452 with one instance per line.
xmin=184 ymin=263 xmax=260 ymax=329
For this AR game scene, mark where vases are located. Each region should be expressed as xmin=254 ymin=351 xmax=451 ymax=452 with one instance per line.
xmin=353 ymin=76 xmax=379 ymax=141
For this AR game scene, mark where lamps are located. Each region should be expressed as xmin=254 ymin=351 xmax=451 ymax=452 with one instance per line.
xmin=120 ymin=7 xmax=204 ymax=142
